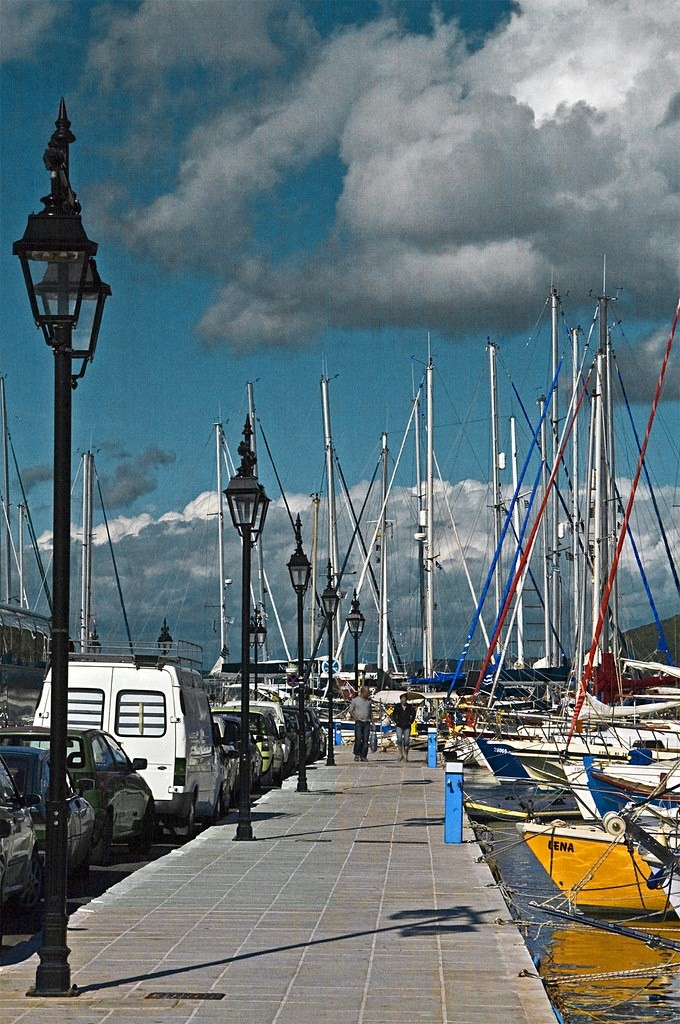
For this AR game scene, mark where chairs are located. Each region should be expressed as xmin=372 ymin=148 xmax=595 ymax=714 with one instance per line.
xmin=68 ymin=752 xmax=83 ymax=766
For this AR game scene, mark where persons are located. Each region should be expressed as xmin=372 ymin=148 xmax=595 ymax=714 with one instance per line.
xmin=392 ymin=694 xmax=416 ymax=764
xmin=348 ymin=686 xmax=375 ymax=763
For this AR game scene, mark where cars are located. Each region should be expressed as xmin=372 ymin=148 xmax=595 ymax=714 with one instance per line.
xmin=0 ymin=754 xmax=46 ymax=915
xmin=0 ymin=746 xmax=97 ymax=889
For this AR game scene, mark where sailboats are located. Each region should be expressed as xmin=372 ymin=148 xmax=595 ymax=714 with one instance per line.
xmin=7 ymin=251 xmax=680 ymax=959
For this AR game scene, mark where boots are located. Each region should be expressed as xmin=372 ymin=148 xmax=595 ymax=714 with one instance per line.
xmin=404 ymin=746 xmax=409 ymax=761
xmin=398 ymin=746 xmax=403 ymax=761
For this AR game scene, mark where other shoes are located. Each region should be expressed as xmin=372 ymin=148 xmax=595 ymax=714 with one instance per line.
xmin=354 ymin=756 xmax=359 ymax=761
xmin=361 ymin=757 xmax=368 ymax=762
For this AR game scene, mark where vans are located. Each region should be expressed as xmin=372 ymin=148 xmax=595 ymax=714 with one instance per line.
xmin=28 ymin=639 xmax=241 ymax=845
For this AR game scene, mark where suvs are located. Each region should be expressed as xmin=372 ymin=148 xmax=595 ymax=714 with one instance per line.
xmin=0 ymin=725 xmax=156 ymax=866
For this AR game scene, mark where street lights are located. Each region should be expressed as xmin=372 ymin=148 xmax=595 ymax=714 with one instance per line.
xmin=345 ymin=587 xmax=367 ymax=697
xmin=249 ymin=605 xmax=268 ymax=701
xmin=223 ymin=412 xmax=275 ymax=843
xmin=9 ymin=93 xmax=113 ymax=995
xmin=285 ymin=512 xmax=313 ymax=793
xmin=319 ymin=557 xmax=341 ymax=767
